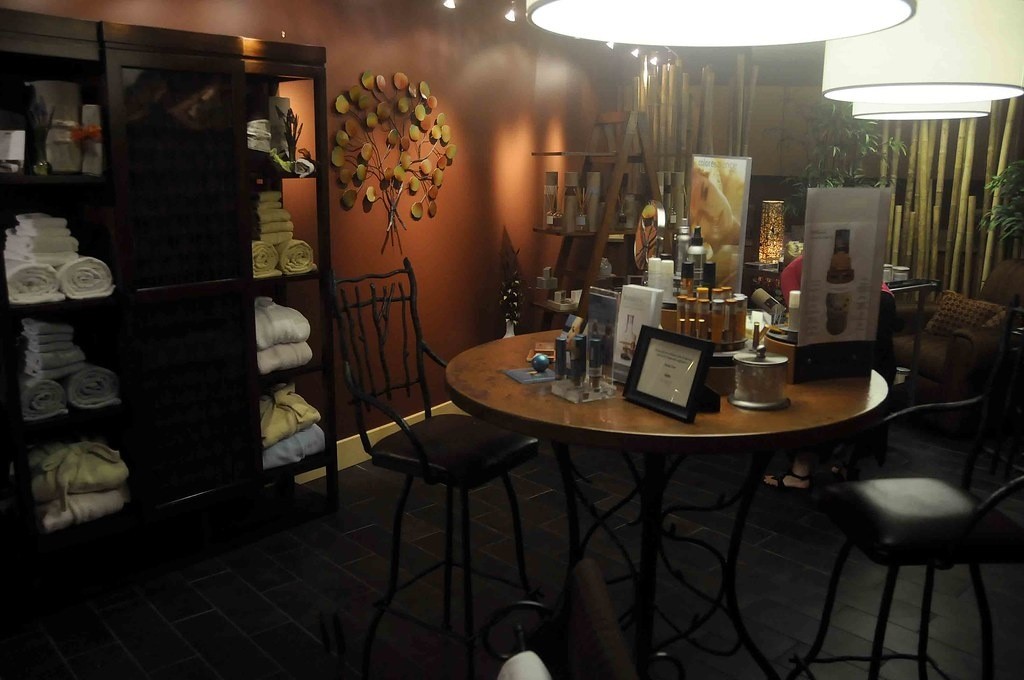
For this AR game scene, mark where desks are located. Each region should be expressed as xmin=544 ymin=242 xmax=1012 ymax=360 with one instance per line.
xmin=744 ymin=261 xmax=942 ymax=368
xmin=445 ymin=326 xmax=888 ymax=680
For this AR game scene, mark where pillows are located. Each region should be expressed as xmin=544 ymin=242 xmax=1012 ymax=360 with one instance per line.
xmin=927 ymin=289 xmax=1006 ymax=337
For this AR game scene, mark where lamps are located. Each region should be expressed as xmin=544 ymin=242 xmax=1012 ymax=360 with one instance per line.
xmin=518 ymin=0 xmax=1024 ymax=121
xmin=760 ymin=198 xmax=785 ymax=264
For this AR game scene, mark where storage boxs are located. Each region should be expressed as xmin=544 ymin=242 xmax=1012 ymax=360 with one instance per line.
xmin=613 ymin=284 xmax=664 ymax=368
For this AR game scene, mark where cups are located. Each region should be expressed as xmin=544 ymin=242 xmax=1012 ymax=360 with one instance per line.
xmin=882 ymin=263 xmax=911 ymax=283
xmin=748 ymin=286 xmax=786 ymax=317
xmin=894 ymin=367 xmax=911 ymax=385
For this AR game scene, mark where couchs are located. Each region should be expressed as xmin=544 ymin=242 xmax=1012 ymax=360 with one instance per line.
xmin=890 ymin=260 xmax=1023 ymax=446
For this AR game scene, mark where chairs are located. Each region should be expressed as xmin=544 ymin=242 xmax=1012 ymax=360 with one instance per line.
xmin=328 ymin=257 xmax=541 ymax=644
xmin=788 ymin=327 xmax=1024 ymax=680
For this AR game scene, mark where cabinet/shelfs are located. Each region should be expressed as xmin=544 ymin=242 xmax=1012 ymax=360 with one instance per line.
xmin=0 ymin=6 xmax=339 ymax=615
xmin=532 ymin=110 xmax=675 ymax=331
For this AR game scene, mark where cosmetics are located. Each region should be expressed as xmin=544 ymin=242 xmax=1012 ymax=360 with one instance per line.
xmin=537 ymin=169 xmax=801 ymax=391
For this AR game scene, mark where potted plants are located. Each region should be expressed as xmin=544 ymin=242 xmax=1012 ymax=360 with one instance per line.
xmin=498 ymin=248 xmax=524 ymax=339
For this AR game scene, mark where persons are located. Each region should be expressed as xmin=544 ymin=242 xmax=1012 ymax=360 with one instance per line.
xmin=692 ymin=159 xmax=742 ymax=254
xmin=760 ymin=255 xmax=897 ymax=490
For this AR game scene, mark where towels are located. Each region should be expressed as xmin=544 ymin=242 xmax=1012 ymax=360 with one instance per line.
xmin=251 ymin=189 xmax=318 ymax=278
xmin=27 ymin=438 xmax=130 ymax=533
xmin=247 ymin=119 xmax=314 ymax=178
xmin=254 ymin=295 xmax=313 ymax=374
xmin=3 ymin=212 xmax=116 ymax=304
xmin=19 ymin=316 xmax=121 ymax=422
xmin=258 ymin=381 xmax=326 ymax=470
